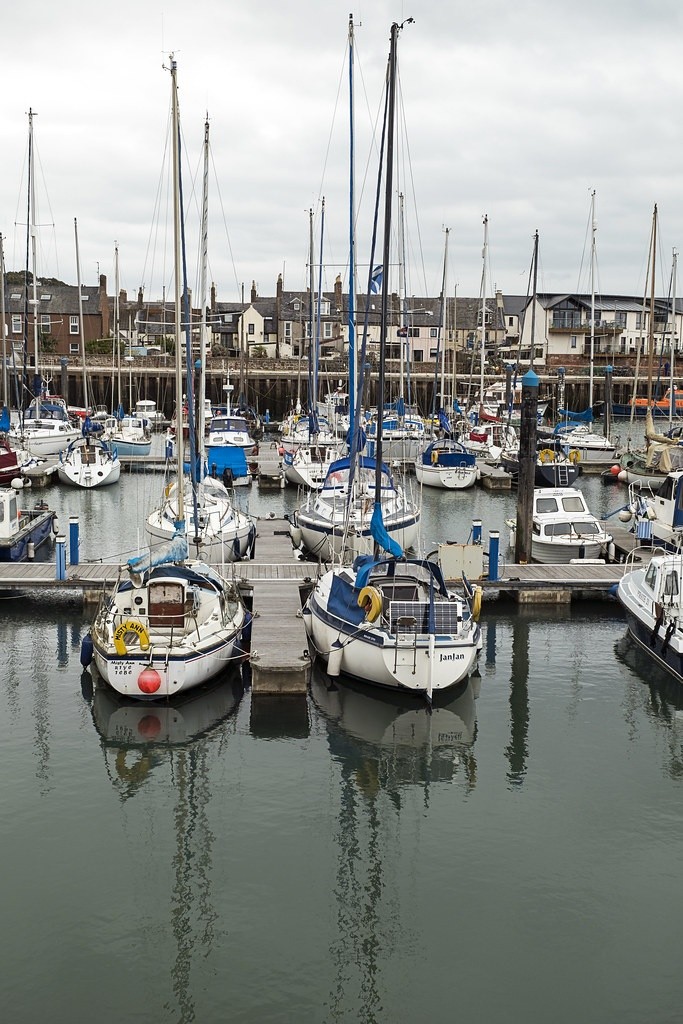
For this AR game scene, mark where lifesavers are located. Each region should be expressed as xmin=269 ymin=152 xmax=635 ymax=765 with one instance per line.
xmin=165 ymin=483 xmax=173 ymax=496
xmin=357 ymin=586 xmax=382 ymax=623
xmin=540 ymin=449 xmax=554 ymax=462
xmin=328 ymin=473 xmax=341 ymax=486
xmin=113 ymin=622 xmax=149 ymax=655
xmin=472 ymin=587 xmax=482 ymax=621
xmin=569 ymin=449 xmax=581 ymax=462
xmin=431 ymin=450 xmax=438 ymax=463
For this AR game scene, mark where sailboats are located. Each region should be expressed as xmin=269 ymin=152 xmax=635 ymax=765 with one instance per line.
xmin=80 ymin=42 xmax=260 ymax=704
xmin=0 ymin=104 xmax=682 ymax=565
xmin=294 ymin=10 xmax=486 ymax=708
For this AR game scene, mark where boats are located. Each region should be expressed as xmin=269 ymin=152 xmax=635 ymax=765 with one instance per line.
xmin=503 ymin=487 xmax=615 ymax=566
xmin=615 ymin=526 xmax=682 ymax=687
xmin=626 ymin=467 xmax=683 ymax=556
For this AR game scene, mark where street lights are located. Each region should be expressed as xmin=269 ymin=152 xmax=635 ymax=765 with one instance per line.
xmin=612 ymin=300 xmax=618 ymax=366
